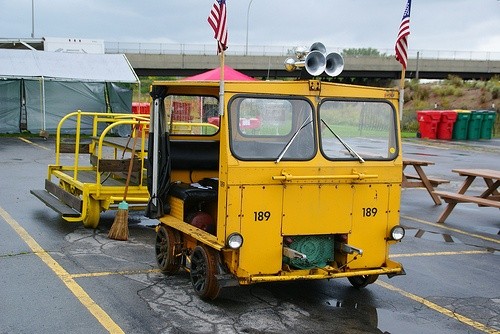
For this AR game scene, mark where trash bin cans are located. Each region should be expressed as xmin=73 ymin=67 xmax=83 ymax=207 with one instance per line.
xmin=170 ymin=101 xmax=191 ymax=131
xmin=415 ymin=109 xmax=496 ymax=141
xmin=132 ymin=104 xmax=151 ymax=135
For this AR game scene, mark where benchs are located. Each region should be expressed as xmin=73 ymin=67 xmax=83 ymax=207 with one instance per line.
xmin=405 ymin=173 xmax=451 ymax=184
xmin=168 ymin=140 xmax=261 ymax=222
xmin=431 ymin=190 xmax=500 ymax=209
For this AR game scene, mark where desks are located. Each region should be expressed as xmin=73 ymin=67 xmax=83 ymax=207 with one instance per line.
xmin=340 ymin=150 xmax=443 ymax=206
xmin=435 ymin=168 xmax=500 ymax=223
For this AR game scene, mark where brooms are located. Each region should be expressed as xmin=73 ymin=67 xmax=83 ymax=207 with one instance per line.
xmin=108 ymin=124 xmax=140 ymax=241
